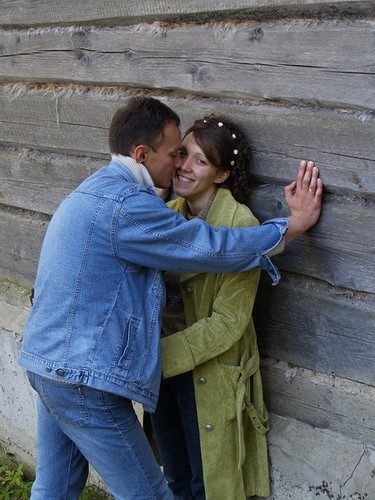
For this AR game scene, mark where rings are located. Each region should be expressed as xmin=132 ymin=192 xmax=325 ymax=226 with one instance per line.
xmin=308 ymin=188 xmax=315 ymax=192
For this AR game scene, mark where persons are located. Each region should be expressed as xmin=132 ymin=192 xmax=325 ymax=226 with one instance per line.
xmin=144 ymin=113 xmax=269 ymax=500
xmin=17 ymin=96 xmax=323 ymax=500
